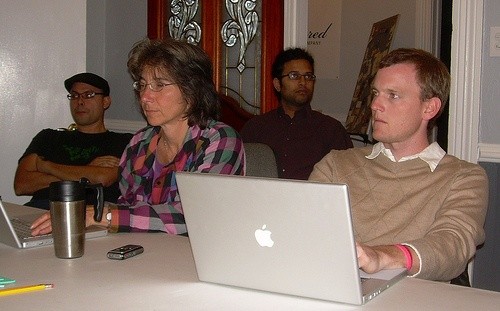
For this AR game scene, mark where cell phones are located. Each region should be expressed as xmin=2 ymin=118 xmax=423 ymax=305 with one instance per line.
xmin=106 ymin=244 xmax=145 ymax=259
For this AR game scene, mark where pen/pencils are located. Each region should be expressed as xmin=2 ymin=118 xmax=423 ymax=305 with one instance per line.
xmin=0 ymin=283 xmax=54 ymax=297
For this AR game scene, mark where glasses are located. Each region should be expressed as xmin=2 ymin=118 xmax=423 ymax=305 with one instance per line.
xmin=67 ymin=90 xmax=105 ymax=100
xmin=280 ymin=71 xmax=316 ymax=82
xmin=131 ymin=80 xmax=178 ymax=91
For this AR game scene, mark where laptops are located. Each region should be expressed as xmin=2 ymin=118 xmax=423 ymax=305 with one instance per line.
xmin=0 ymin=200 xmax=109 ymax=249
xmin=175 ymin=172 xmax=408 ymax=306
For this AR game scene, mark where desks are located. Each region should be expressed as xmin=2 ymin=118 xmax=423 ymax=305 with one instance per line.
xmin=0 ymin=202 xmax=500 ymax=311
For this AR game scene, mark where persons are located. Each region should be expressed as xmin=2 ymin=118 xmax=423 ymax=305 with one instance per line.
xmin=309 ymin=48 xmax=490 ymax=285
xmin=29 ymin=39 xmax=246 ymax=237
xmin=14 ymin=73 xmax=136 ymax=210
xmin=238 ymin=48 xmax=355 ymax=180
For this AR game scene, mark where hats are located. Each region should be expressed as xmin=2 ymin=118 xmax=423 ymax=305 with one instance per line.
xmin=64 ymin=73 xmax=110 ymax=97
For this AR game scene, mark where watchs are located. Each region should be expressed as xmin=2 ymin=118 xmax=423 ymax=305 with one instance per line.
xmin=106 ymin=207 xmax=111 ymax=226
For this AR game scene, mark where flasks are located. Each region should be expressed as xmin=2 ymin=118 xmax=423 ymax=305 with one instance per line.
xmin=48 ymin=177 xmax=105 ymax=260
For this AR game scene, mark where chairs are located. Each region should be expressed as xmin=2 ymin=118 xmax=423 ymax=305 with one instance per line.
xmin=244 ymin=143 xmax=278 ymax=178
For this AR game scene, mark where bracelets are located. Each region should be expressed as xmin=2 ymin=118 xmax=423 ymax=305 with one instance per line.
xmin=394 ymin=243 xmax=413 ymax=272
